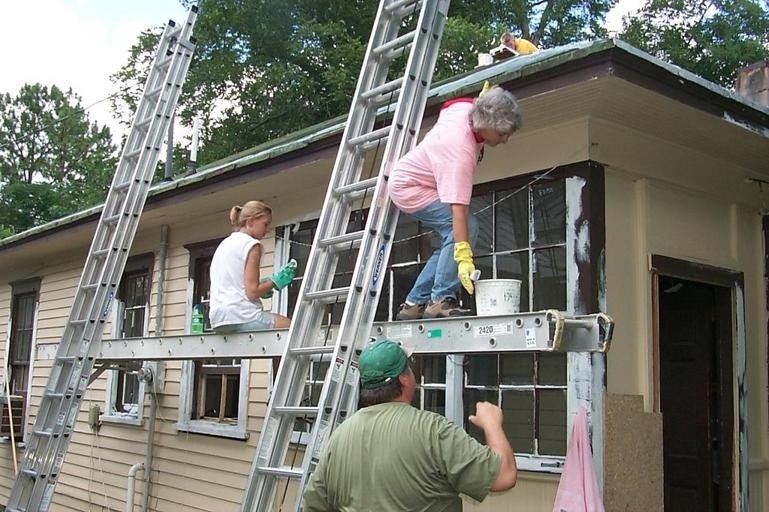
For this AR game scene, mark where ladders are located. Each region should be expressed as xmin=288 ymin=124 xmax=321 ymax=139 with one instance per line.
xmin=6 ymin=6 xmax=197 ymax=512
xmin=246 ymin=1 xmax=450 ymax=512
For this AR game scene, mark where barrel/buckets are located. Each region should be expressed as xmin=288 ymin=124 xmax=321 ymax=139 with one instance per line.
xmin=474 ymin=279 xmax=522 ymax=318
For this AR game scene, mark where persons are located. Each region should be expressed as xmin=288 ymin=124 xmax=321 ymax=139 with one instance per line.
xmin=385 ymin=79 xmax=524 ymax=321
xmin=498 ymin=33 xmax=540 ymax=55
xmin=206 ymin=199 xmax=297 ymax=333
xmin=301 ymin=340 xmax=519 ymax=512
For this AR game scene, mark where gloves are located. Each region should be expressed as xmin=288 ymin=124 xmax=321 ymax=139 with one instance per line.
xmin=454 ymin=242 xmax=481 ymax=295
xmin=260 ymin=259 xmax=297 ymax=299
xmin=477 ymin=80 xmax=491 ymax=96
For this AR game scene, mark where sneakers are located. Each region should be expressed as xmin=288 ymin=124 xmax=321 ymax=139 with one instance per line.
xmin=298 ymin=399 xmax=314 ymax=424
xmin=397 ymin=299 xmax=474 ymax=319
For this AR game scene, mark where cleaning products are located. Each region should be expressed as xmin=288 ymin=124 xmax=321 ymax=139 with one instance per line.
xmin=190 ymin=304 xmax=205 ymax=334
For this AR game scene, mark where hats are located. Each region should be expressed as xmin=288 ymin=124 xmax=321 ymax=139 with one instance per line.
xmin=357 ymin=339 xmax=413 ymax=389
